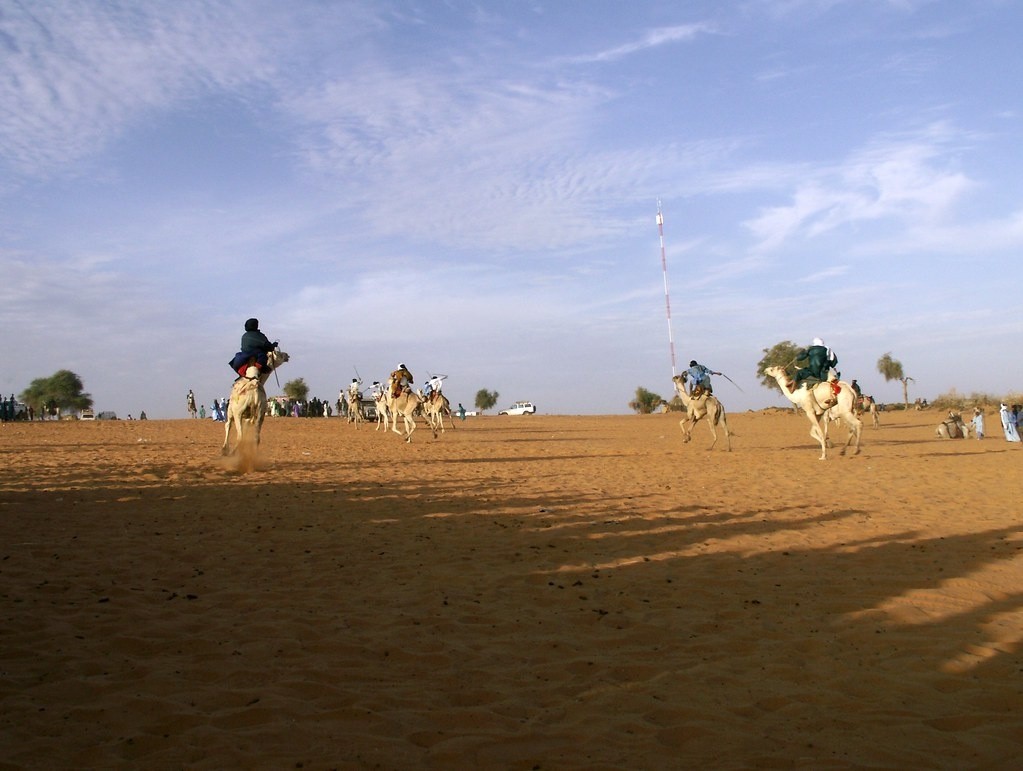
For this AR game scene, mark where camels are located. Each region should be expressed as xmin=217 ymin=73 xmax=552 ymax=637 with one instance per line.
xmin=936 ymin=406 xmax=984 ymax=440
xmin=346 ymin=389 xmax=363 ymax=429
xmin=370 ymin=377 xmax=456 ymax=443
xmin=223 ymin=348 xmax=290 ymax=457
xmin=764 ymin=365 xmax=879 ymax=459
xmin=672 ymin=375 xmax=731 ymax=452
xmin=186 ymin=394 xmax=197 ymax=419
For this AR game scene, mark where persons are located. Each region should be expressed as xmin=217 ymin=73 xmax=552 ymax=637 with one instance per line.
xmin=267 ymin=397 xmax=331 ymax=417
xmin=0 ymin=393 xmax=33 ymax=422
xmin=200 ymin=405 xmax=206 ymax=418
xmin=339 ymin=390 xmax=345 ymax=402
xmin=50 ymin=408 xmax=57 ymax=419
xmin=210 ymin=398 xmax=229 ymax=422
xmin=971 ymin=403 xmax=1023 ymax=442
xmin=458 ymin=404 xmax=466 ymax=421
xmin=335 ymin=399 xmax=342 ymax=416
xmin=187 ymin=390 xmax=193 ymax=405
xmin=788 ymin=338 xmax=839 ymax=390
xmin=852 ymin=380 xmax=860 ymax=394
xmin=423 ymin=376 xmax=446 ymax=397
xmin=127 ymin=415 xmax=132 ymax=420
xmin=834 ymin=368 xmax=841 ymax=379
xmin=681 ymin=360 xmax=722 ymax=397
xmin=341 ymin=396 xmax=348 ymax=418
xmin=368 ymin=382 xmax=385 ymax=394
xmin=141 ymin=411 xmax=146 ymax=419
xmin=391 ymin=363 xmax=413 ymax=398
xmin=229 ymin=318 xmax=278 ymax=377
xmin=351 ymin=378 xmax=363 ymax=403
xmin=922 ymin=399 xmax=927 ymax=407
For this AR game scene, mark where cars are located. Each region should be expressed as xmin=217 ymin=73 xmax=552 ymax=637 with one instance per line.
xmin=81 ymin=414 xmax=95 ymax=421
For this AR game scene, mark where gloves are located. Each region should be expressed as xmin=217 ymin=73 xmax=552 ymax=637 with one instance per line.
xmin=273 ymin=342 xmax=278 ymax=347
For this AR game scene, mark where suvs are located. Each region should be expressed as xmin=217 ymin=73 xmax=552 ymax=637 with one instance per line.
xmin=496 ymin=402 xmax=538 ymax=417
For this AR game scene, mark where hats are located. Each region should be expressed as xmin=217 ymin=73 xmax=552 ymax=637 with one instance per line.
xmin=245 ymin=318 xmax=259 ymax=332
xmin=690 ymin=360 xmax=697 ymax=367
